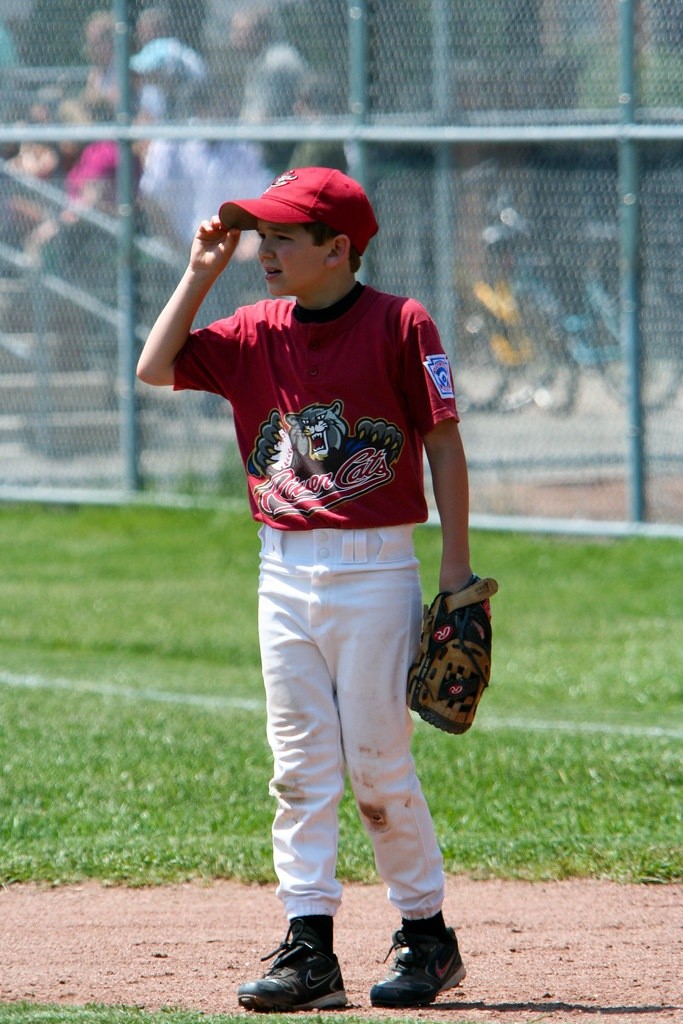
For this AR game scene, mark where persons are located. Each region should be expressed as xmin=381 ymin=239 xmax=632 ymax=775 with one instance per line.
xmin=1 ymin=9 xmax=343 ymax=368
xmin=136 ymin=166 xmax=499 ymax=1010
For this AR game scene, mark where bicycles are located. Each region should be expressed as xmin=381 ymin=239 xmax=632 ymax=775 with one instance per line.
xmin=412 ymin=148 xmax=683 ymax=414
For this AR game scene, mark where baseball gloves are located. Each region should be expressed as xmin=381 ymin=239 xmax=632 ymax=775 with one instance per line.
xmin=404 ymin=574 xmax=499 ymax=737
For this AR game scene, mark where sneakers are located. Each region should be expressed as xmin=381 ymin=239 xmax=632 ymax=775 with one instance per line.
xmin=237 ymin=917 xmax=348 ymax=1009
xmin=369 ymin=924 xmax=466 ymax=1008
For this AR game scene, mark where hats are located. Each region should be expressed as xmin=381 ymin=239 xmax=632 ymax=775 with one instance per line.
xmin=221 ymin=166 xmax=379 ymax=255
